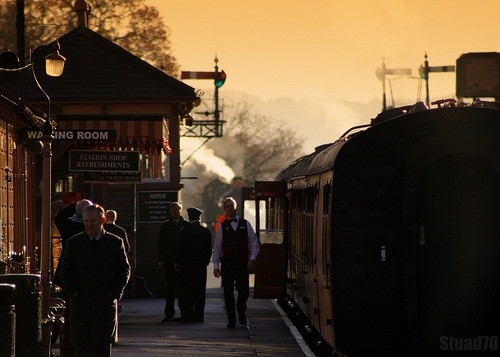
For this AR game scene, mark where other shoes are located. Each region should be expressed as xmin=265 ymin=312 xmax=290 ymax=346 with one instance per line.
xmin=161 ymin=318 xmax=172 ymax=322
xmin=227 ymin=323 xmax=235 ymax=328
xmin=238 ymin=312 xmax=246 ymax=325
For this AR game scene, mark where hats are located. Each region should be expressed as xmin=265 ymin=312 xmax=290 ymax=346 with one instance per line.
xmin=187 ymin=207 xmax=202 ymax=216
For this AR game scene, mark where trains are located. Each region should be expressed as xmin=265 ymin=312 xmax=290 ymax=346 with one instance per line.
xmin=241 ymin=52 xmax=500 ymax=356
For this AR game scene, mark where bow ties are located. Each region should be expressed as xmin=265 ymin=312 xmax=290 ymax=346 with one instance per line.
xmin=228 ymin=218 xmax=238 ymax=223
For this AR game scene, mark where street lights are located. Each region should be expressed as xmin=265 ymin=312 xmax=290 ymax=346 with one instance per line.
xmin=40 ymin=38 xmax=66 ymax=356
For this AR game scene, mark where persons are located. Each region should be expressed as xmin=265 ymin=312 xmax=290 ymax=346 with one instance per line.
xmin=54 ymin=199 xmax=94 ymax=262
xmin=157 ymin=202 xmax=189 ymax=323
xmin=52 ymin=205 xmax=131 ymax=357
xmin=213 ymin=197 xmax=260 ymax=328
xmin=102 ymin=209 xmax=131 ymax=312
xmin=178 ymin=207 xmax=212 ymax=323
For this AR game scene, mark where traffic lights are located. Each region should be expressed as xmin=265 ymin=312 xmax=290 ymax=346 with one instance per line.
xmin=214 ymin=70 xmax=226 ymax=88
